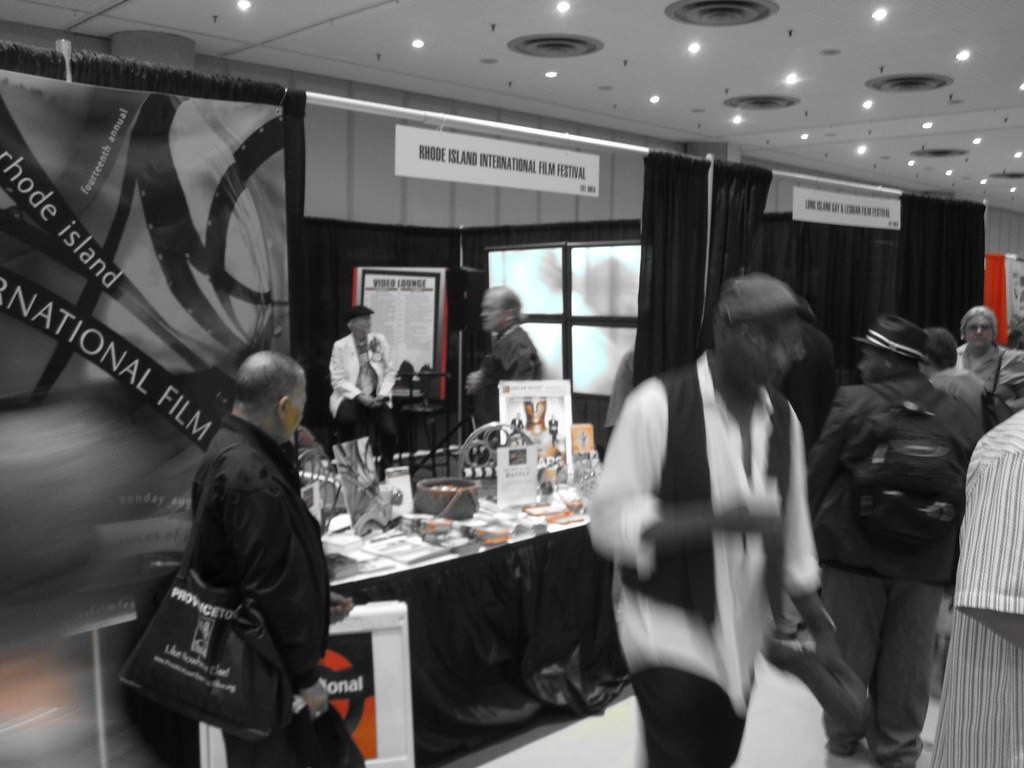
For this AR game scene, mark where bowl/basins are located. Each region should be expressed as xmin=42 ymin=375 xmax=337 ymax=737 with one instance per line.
xmin=416 ymin=477 xmax=478 ymax=519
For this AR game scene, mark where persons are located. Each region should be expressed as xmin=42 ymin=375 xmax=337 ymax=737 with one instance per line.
xmin=329 ymin=306 xmax=397 ymax=457
xmin=191 ymin=350 xmax=366 ymax=768
xmin=588 ymin=273 xmax=1023 ymax=768
xmin=467 ymin=287 xmax=542 ymax=442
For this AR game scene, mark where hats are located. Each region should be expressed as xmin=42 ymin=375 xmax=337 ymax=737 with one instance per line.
xmin=853 ymin=312 xmax=933 ymax=367
xmin=711 ymin=271 xmax=817 ymax=326
xmin=345 ymin=305 xmax=374 ymax=321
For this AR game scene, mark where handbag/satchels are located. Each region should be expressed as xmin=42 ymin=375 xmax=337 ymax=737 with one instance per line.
xmin=984 ymin=352 xmax=1015 ymax=430
xmin=118 ymin=443 xmax=294 ymax=743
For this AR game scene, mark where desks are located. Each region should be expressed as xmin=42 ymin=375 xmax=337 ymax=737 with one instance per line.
xmin=136 ymin=502 xmax=639 ymax=768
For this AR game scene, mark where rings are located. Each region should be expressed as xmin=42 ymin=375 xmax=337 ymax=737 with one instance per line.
xmin=315 ymin=712 xmax=321 ymax=717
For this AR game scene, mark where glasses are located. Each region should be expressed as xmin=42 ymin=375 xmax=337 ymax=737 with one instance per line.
xmin=965 ymin=323 xmax=992 ymax=332
